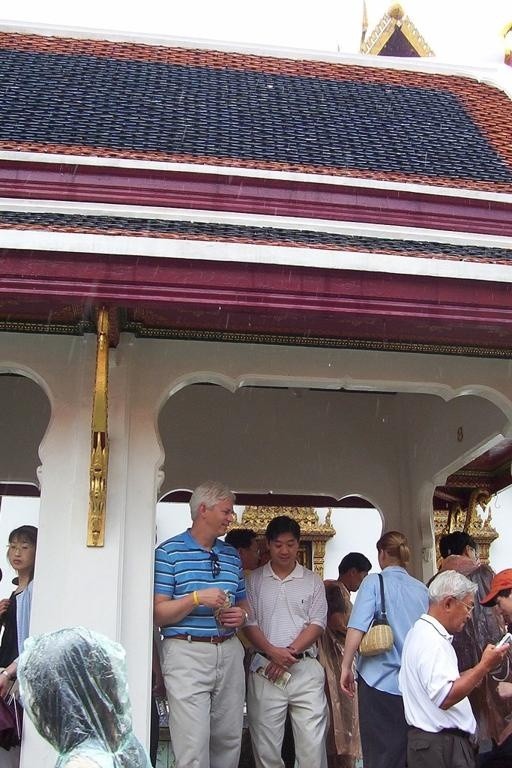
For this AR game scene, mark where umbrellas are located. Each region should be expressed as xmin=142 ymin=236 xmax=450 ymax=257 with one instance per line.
xmin=0 ymin=679 xmax=23 ymax=752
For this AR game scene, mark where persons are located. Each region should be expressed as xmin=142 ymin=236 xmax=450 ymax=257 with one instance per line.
xmin=0 ymin=524 xmax=38 ymax=699
xmin=17 ymin=625 xmax=154 ymax=768
xmin=151 ymin=479 xmax=511 ymax=767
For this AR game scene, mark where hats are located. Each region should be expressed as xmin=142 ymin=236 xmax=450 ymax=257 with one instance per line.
xmin=479 ymin=568 xmax=512 ymax=607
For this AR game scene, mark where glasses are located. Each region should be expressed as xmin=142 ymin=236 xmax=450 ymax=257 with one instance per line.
xmin=453 ymin=598 xmax=475 ymax=614
xmin=209 ymin=554 xmax=221 ymax=579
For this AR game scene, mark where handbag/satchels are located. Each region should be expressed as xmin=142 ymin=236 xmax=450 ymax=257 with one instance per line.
xmin=356 ymin=617 xmax=394 ymax=657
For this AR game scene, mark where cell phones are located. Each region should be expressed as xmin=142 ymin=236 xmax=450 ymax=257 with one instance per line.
xmin=496 ymin=633 xmax=512 ymax=649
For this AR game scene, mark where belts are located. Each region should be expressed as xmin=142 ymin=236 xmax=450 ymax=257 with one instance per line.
xmin=165 ymin=633 xmax=235 ymax=643
xmin=255 ymin=651 xmax=310 ymax=660
xmin=440 ymin=728 xmax=471 ymax=738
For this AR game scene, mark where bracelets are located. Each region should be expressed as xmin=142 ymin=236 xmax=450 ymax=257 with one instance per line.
xmin=1 ymin=668 xmax=17 ymax=684
